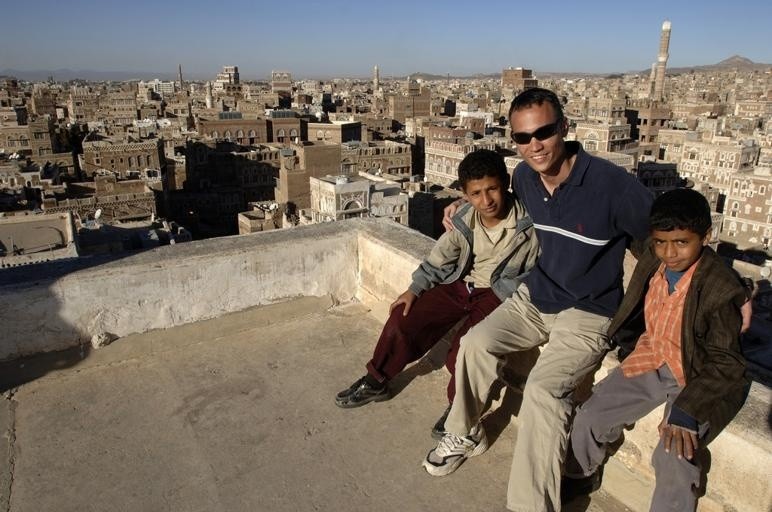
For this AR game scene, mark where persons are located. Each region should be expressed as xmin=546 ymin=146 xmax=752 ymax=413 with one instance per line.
xmin=562 ymin=186 xmax=752 ymax=512
xmin=421 ymin=85 xmax=753 ymax=512
xmin=334 ymin=148 xmax=543 ymax=437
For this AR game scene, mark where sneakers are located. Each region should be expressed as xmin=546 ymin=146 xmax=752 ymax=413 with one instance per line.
xmin=432 ymin=404 xmax=453 ymax=437
xmin=334 ymin=376 xmax=391 ymax=408
xmin=422 ymin=424 xmax=489 ymax=477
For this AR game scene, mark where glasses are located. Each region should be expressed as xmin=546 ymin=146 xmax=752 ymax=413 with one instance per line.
xmin=511 ymin=122 xmax=560 ymax=144
